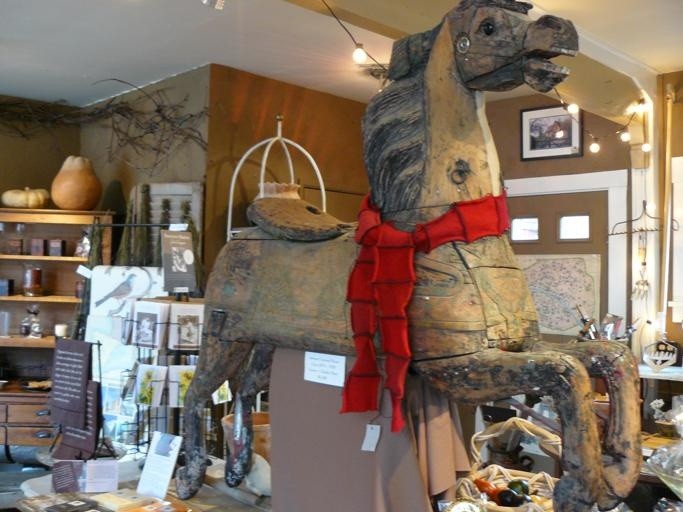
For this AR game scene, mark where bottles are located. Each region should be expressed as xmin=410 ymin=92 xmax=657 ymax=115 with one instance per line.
xmin=20 ymin=304 xmax=42 ymax=338
xmin=20 ymin=267 xmax=42 ymax=298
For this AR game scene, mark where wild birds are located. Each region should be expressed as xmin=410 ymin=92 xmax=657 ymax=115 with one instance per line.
xmin=95 ymin=274 xmax=138 ymax=307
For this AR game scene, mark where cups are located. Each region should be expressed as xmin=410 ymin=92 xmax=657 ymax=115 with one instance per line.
xmin=75 ymin=280 xmax=85 ymax=297
xmin=53 ymin=323 xmax=70 ymax=337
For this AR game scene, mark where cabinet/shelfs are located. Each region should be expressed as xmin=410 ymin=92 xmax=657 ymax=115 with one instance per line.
xmin=0 ymin=208 xmax=116 ymax=448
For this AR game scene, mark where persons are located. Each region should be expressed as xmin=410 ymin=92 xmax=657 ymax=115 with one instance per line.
xmin=139 ymin=314 xmax=153 ymax=340
xmin=184 ymin=320 xmax=195 ymax=341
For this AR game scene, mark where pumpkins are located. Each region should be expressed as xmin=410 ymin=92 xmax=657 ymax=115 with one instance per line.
xmin=2 ymin=184 xmax=49 ymax=209
xmin=50 ymin=155 xmax=102 ymax=211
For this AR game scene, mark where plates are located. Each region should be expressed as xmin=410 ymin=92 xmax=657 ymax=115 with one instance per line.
xmin=20 ymin=382 xmax=51 ymax=392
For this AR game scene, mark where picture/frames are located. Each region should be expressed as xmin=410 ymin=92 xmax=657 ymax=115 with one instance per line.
xmin=519 ymin=103 xmax=584 ymax=161
